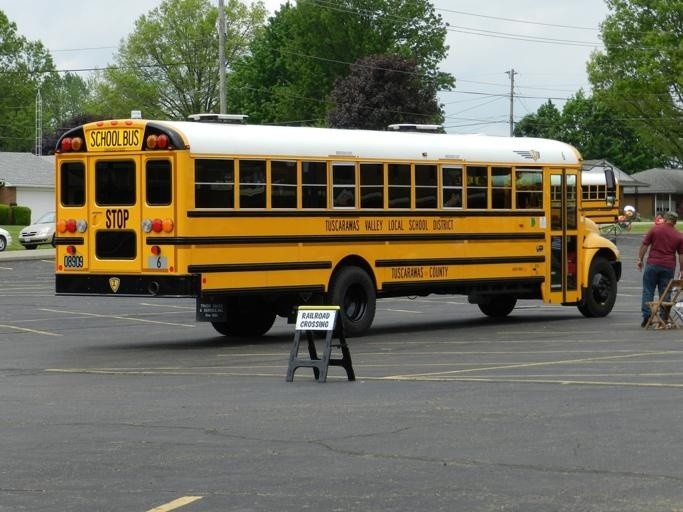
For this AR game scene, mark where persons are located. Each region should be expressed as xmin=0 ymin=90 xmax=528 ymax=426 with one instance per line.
xmin=636 ymin=211 xmax=683 ymax=328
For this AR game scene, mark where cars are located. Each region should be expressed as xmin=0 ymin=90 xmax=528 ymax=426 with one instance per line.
xmin=0 ymin=228 xmax=12 ymax=251
xmin=18 ymin=210 xmax=56 ymax=249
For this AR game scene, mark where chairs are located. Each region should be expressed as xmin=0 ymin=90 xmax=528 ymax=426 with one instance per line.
xmin=643 ymin=277 xmax=682 ymax=331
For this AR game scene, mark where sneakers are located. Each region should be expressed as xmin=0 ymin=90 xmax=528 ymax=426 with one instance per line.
xmin=641 ymin=316 xmax=653 ymax=327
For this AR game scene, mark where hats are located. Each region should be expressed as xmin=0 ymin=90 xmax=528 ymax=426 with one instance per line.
xmin=664 ymin=212 xmax=678 ymax=220
